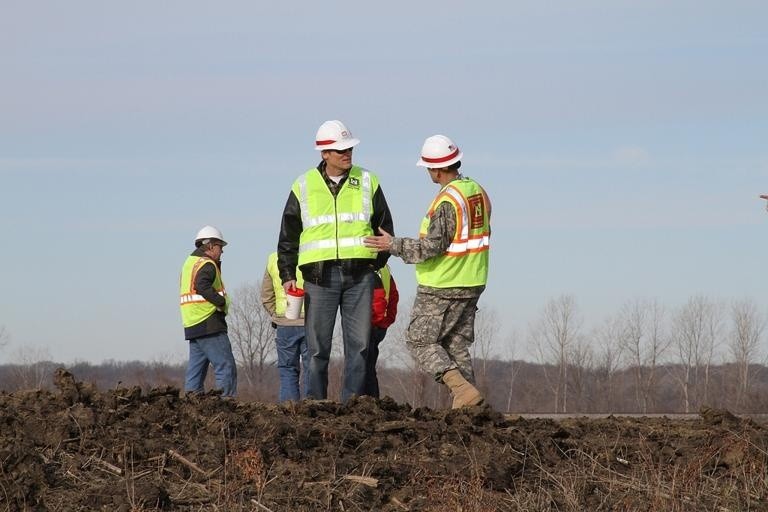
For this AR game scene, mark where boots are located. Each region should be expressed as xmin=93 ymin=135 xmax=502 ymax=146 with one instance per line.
xmin=443 ymin=369 xmax=483 ymax=409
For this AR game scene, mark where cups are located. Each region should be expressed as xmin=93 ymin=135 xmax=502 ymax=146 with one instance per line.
xmin=285 ymin=287 xmax=305 ymax=319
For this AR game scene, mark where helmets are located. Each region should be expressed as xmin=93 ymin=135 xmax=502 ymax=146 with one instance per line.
xmin=315 ymin=121 xmax=359 ymax=151
xmin=416 ymin=135 xmax=464 ymax=169
xmin=195 ymin=225 xmax=226 ymax=247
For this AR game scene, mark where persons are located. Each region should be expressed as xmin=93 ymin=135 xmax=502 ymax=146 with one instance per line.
xmin=365 ymin=263 xmax=399 ymax=398
xmin=261 ymin=252 xmax=309 ymax=403
xmin=179 ymin=225 xmax=237 ymax=400
xmin=277 ymin=120 xmax=394 ymax=404
xmin=363 ymin=134 xmax=491 ymax=410
